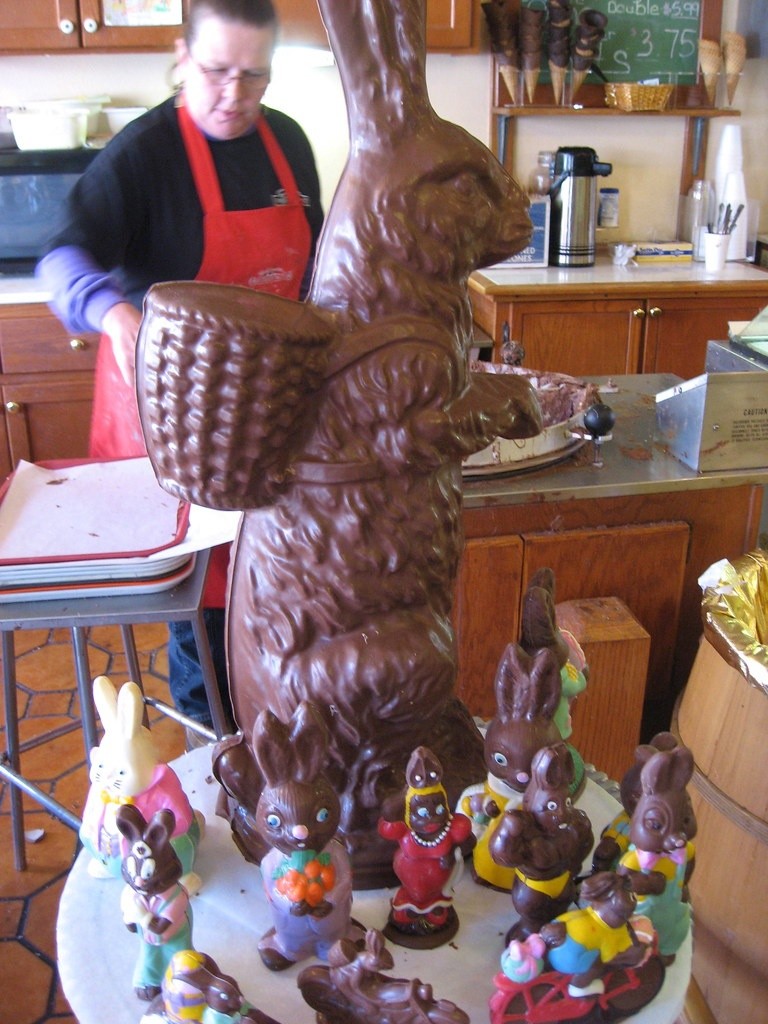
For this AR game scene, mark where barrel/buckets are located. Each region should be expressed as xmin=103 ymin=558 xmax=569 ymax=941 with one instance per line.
xmin=669 ymin=547 xmax=768 ymax=1024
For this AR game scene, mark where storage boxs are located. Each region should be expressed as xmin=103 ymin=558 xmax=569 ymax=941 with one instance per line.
xmin=0 ymin=97 xmax=151 ymax=152
xmin=634 ymin=240 xmax=694 ymax=263
xmin=484 ymin=193 xmax=550 ymax=269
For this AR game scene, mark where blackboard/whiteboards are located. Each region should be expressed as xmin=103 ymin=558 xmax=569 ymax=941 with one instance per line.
xmin=522 ymin=0 xmax=702 ymax=85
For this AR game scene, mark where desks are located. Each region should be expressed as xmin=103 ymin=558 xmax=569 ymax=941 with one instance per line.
xmin=55 ymin=724 xmax=693 ymax=1024
xmin=1 ymin=548 xmax=228 ymax=872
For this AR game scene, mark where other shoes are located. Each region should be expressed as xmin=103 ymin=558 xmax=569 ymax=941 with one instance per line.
xmin=185 ymin=723 xmax=234 ymax=753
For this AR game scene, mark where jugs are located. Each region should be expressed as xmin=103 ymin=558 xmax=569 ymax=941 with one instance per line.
xmin=546 ymin=148 xmax=611 ymax=268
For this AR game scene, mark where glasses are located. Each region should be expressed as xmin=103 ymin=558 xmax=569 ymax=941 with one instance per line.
xmin=185 ymin=40 xmax=274 ymax=90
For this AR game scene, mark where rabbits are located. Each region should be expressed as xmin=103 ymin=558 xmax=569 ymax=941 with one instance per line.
xmin=209 ymin=0 xmax=544 ymax=892
xmin=78 ymin=567 xmax=699 ymax=1024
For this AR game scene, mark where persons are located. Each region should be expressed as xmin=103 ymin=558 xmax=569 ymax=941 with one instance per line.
xmin=32 ymin=0 xmax=325 ymax=459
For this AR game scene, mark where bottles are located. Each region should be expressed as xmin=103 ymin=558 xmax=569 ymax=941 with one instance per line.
xmin=685 ymin=179 xmax=716 ymax=263
xmin=528 ymin=151 xmax=559 ymax=195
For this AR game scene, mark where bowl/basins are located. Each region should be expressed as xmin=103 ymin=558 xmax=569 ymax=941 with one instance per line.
xmin=8 ymin=97 xmax=151 ymax=151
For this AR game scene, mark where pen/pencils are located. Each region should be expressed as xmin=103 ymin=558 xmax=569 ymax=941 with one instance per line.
xmin=705 ymin=201 xmax=745 ymax=234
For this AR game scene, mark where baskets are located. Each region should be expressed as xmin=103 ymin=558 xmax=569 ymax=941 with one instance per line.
xmin=605 ymin=82 xmax=674 ymax=112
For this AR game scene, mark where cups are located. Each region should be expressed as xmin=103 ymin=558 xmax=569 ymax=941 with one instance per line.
xmin=711 ymin=123 xmax=748 ymax=260
xmin=702 ymin=232 xmax=730 ymax=266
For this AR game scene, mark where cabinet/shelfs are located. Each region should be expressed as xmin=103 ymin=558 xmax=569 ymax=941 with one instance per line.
xmin=467 ymin=0 xmax=768 ymax=381
xmin=0 ymin=0 xmax=481 ymax=55
xmin=1 ymin=304 xmax=99 ymax=483
